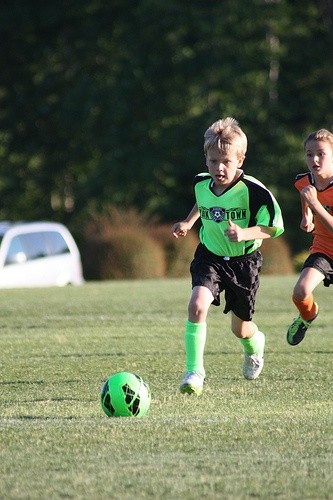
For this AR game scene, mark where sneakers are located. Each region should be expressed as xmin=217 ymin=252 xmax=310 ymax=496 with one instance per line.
xmin=287 ymin=302 xmax=319 ymax=346
xmin=243 ymin=332 xmax=265 ymax=380
xmin=179 ymin=370 xmax=205 ymax=395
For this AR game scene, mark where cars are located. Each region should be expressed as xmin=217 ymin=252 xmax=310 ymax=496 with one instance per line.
xmin=0 ymin=220 xmax=86 ymax=289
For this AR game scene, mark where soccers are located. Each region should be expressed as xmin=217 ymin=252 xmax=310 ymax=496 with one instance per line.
xmin=100 ymin=371 xmax=153 ymax=421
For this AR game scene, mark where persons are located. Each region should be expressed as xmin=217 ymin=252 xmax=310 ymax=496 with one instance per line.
xmin=287 ymin=130 xmax=333 ymax=346
xmin=171 ymin=117 xmax=284 ymax=394
